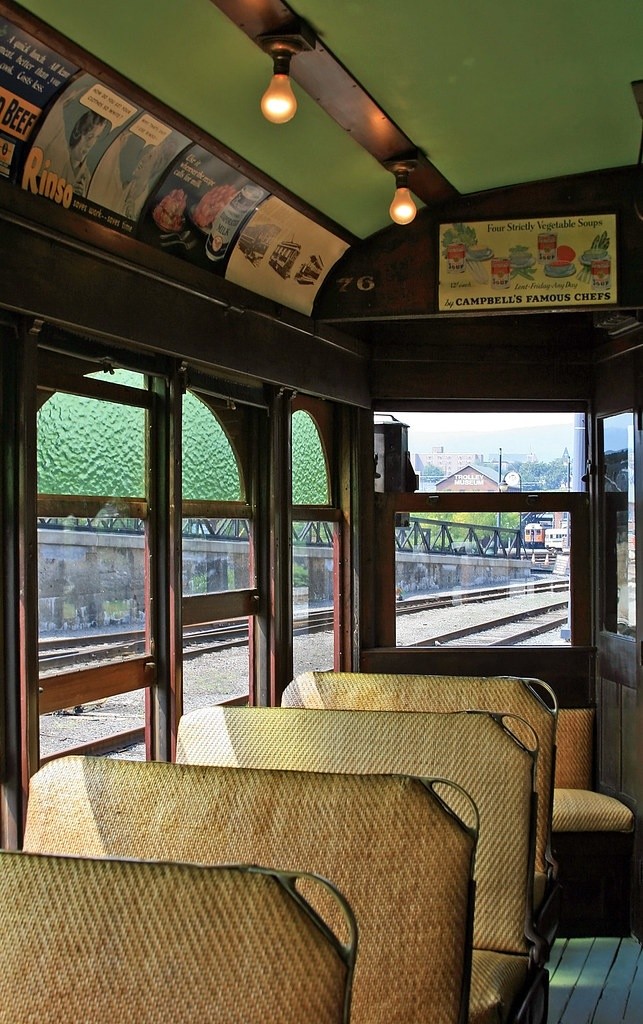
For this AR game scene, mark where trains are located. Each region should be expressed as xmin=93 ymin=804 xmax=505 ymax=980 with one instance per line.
xmin=545 ymin=529 xmax=571 ymax=549
xmin=524 ymin=523 xmax=545 ymax=548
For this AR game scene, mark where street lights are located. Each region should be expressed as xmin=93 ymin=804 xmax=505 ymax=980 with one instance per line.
xmin=498 ymin=471 xmax=521 ymax=529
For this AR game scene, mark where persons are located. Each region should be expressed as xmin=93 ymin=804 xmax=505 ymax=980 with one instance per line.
xmin=87 ymin=110 xmax=165 ymax=216
xmin=56 ymin=109 xmax=108 ymax=199
xmin=123 ymin=136 xmax=179 ymax=222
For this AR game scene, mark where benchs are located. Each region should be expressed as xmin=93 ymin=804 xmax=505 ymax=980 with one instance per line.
xmin=0 ymin=671 xmax=632 ymax=1024
